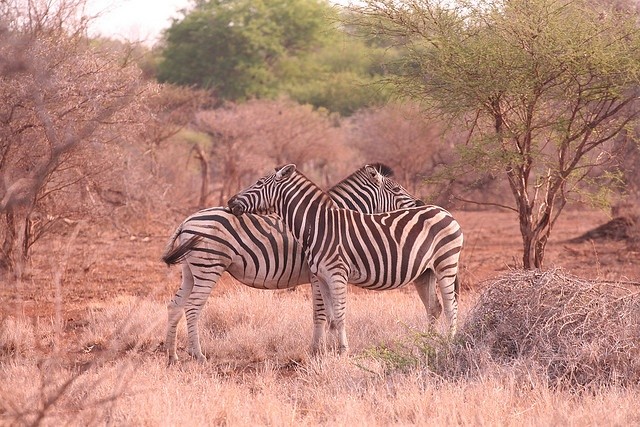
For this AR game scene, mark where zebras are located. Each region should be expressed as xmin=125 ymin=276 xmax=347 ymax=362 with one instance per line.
xmin=158 ymin=162 xmax=426 ymax=367
xmin=227 ymin=164 xmax=463 ymax=354
